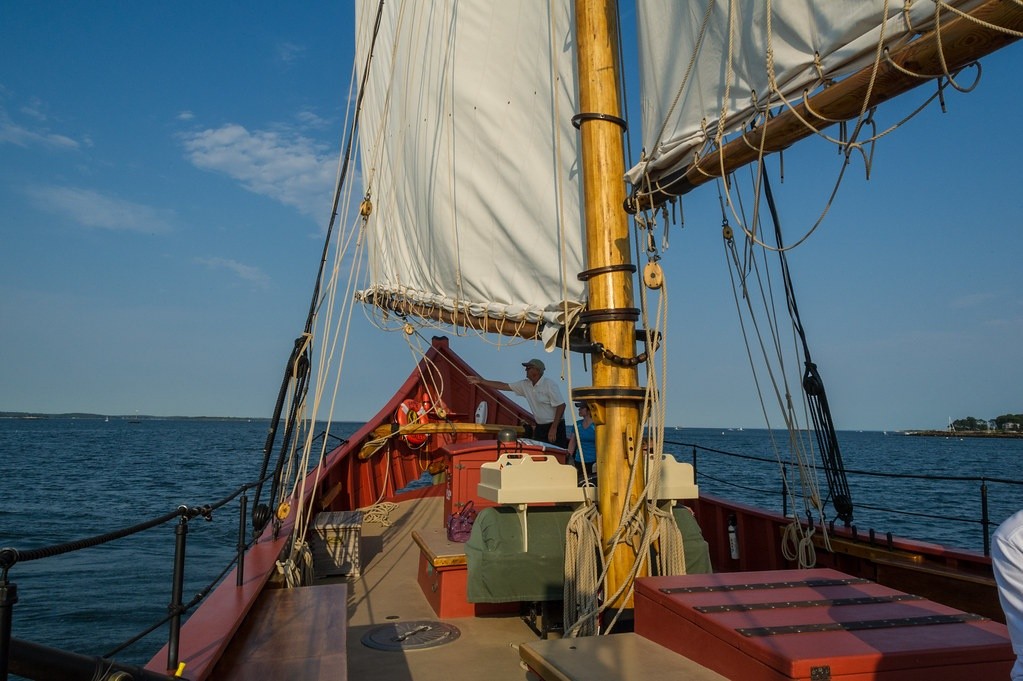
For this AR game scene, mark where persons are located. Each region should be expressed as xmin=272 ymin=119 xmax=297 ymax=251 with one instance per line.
xmin=467 ymin=359 xmax=569 ymax=448
xmin=568 ymin=401 xmax=598 ymax=487
xmin=991 ymin=507 xmax=1023 ymax=681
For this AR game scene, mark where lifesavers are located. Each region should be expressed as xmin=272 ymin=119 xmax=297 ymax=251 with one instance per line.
xmin=395 ymin=399 xmax=431 ymax=444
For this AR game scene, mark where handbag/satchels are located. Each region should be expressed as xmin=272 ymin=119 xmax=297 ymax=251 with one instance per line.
xmin=447 ymin=500 xmax=479 ymax=544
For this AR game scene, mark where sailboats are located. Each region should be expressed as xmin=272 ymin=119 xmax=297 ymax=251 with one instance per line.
xmin=1 ymin=1 xmax=1023 ymax=681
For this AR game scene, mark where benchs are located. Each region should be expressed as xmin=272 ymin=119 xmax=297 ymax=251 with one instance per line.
xmin=206 ymin=581 xmax=349 ymax=681
xmin=518 ymin=630 xmax=731 ymax=681
xmin=411 ymin=525 xmax=478 ymax=617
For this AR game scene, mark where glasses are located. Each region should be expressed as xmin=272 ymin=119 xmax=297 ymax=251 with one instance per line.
xmin=524 ymin=367 xmax=538 ymax=371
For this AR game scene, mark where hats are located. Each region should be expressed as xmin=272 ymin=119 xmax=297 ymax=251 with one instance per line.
xmin=574 ymin=401 xmax=588 ymax=407
xmin=522 ymin=359 xmax=545 ymax=372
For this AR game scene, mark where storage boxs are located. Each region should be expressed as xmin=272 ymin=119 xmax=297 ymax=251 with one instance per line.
xmin=309 ymin=510 xmax=365 ymax=578
